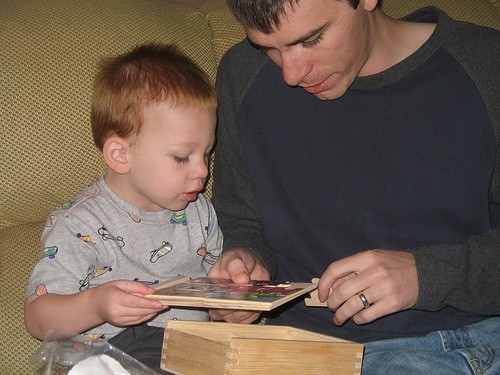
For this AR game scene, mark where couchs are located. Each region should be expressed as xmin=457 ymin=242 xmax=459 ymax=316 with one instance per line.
xmin=0 ymin=0 xmax=500 ymax=375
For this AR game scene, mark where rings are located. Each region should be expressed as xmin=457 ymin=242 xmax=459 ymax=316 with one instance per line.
xmin=358 ymin=292 xmax=369 ymax=308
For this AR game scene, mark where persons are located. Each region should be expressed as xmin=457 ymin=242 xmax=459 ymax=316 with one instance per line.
xmin=202 ymin=0 xmax=500 ymax=375
xmin=23 ymin=40 xmax=224 ymax=375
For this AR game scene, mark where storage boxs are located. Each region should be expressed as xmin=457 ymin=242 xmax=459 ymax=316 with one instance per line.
xmin=159 ymin=319 xmax=364 ymax=375
